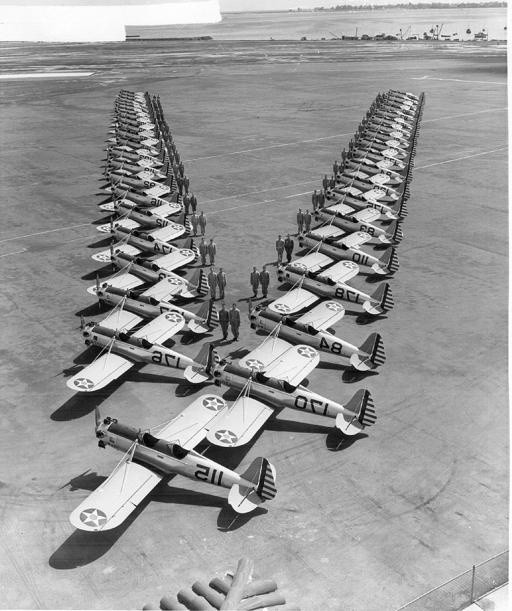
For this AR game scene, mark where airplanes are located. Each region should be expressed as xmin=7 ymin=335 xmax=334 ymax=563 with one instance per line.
xmin=68 ymin=392 xmax=277 ymax=532
